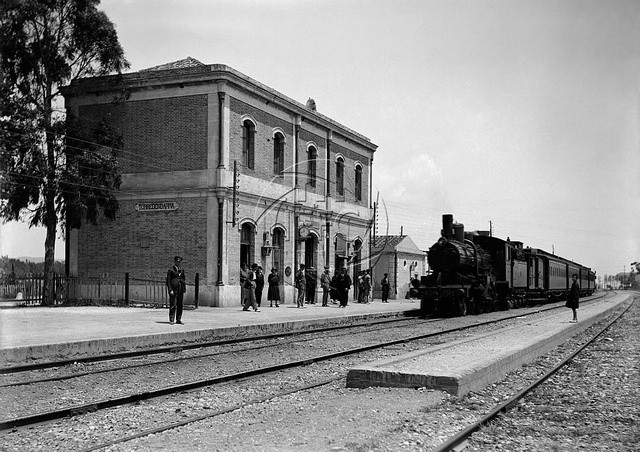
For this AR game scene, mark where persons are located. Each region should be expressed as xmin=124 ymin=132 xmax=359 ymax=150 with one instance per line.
xmin=295 ymin=264 xmax=307 ymax=308
xmin=364 ymin=270 xmax=372 ymax=304
xmin=339 ymin=268 xmax=352 ymax=307
xmin=321 ymin=266 xmax=331 ymax=306
xmin=381 ymin=273 xmax=391 ymax=303
xmin=165 ymin=256 xmax=186 ymax=325
xmin=565 ymin=273 xmax=579 ymax=323
xmin=239 ymin=263 xmax=249 ymax=305
xmin=243 ymin=264 xmax=261 ymax=312
xmin=358 ymin=271 xmax=364 ymax=302
xmin=256 ymin=267 xmax=265 ymax=306
xmin=307 ymin=266 xmax=317 ymax=304
xmin=267 ymin=267 xmax=280 ymax=307
xmin=331 ymin=269 xmax=338 ymax=304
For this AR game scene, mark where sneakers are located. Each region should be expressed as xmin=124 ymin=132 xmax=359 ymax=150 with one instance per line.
xmin=569 ymin=320 xmax=576 ymax=323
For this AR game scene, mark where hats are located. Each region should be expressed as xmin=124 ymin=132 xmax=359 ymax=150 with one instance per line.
xmin=174 ymin=256 xmax=183 ymax=260
xmin=271 ymin=267 xmax=277 ymax=272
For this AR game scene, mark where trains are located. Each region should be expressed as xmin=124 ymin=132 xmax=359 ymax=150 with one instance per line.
xmin=410 ymin=214 xmax=597 ymax=318
